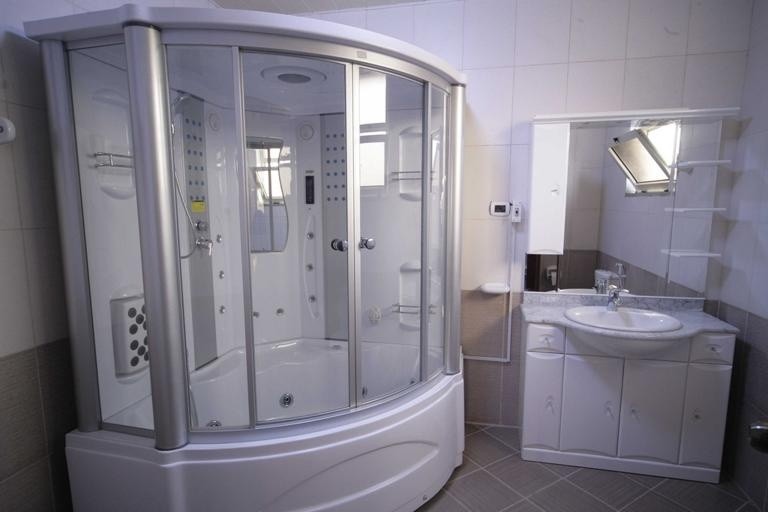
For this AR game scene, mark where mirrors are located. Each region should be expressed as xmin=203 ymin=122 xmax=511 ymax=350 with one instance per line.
xmin=246 ymin=136 xmax=290 ymax=253
xmin=529 ymin=113 xmax=724 ymax=299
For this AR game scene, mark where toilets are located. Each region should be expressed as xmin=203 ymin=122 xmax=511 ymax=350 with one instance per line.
xmin=594 ymin=270 xmax=625 ymax=287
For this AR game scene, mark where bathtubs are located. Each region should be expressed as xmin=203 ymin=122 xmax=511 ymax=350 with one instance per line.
xmin=65 ymin=338 xmax=461 ymax=467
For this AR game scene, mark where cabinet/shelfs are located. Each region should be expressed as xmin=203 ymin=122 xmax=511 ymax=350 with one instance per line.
xmin=525 ymin=325 xmax=735 ymax=471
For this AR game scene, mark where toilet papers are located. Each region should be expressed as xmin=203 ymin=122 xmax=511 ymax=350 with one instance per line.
xmin=551 ymin=272 xmax=556 ymax=286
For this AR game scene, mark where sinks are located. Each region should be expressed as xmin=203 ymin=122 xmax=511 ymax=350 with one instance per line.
xmin=547 ymin=288 xmax=631 ymax=295
xmin=564 ymin=307 xmax=683 ymax=332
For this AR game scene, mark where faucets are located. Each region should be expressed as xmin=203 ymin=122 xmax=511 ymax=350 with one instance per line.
xmin=592 ymin=279 xmax=607 ymax=294
xmin=608 ymin=285 xmax=624 ymax=311
